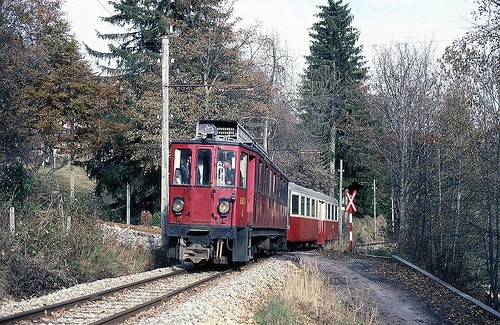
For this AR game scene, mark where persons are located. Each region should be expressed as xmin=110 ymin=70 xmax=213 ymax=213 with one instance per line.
xmin=178 ymin=154 xmax=201 ymax=186
xmin=222 ymin=158 xmax=244 ymax=187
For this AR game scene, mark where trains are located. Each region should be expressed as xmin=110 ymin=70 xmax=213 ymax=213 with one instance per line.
xmin=166 ymin=119 xmax=339 ymax=270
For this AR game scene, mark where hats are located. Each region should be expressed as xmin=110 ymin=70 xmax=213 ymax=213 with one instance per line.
xmin=222 ymin=158 xmax=232 ymax=163
